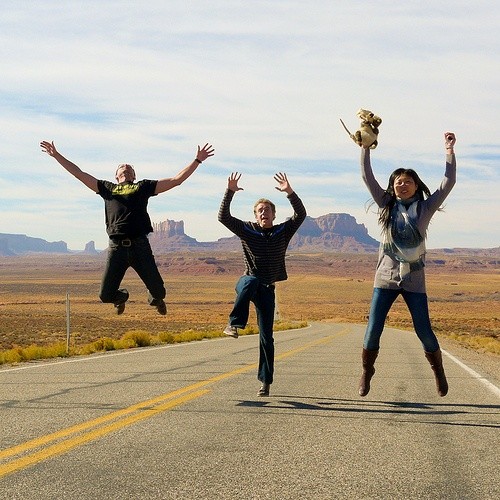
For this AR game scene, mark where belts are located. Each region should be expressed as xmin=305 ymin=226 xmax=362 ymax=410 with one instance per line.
xmin=263 ymin=282 xmax=276 ymax=289
xmin=120 ymin=238 xmax=145 ymax=247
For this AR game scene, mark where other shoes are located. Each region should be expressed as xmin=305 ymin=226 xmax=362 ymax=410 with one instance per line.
xmin=114 ymin=303 xmax=125 ymax=315
xmin=154 ymin=300 xmax=166 ymax=315
xmin=223 ymin=325 xmax=238 ymax=338
xmin=257 ymin=384 xmax=269 ymax=397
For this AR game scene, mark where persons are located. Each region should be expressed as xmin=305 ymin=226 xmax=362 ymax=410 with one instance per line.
xmin=359 ymin=132 xmax=456 ymax=398
xmin=217 ymin=171 xmax=307 ymax=396
xmin=40 ymin=140 xmax=215 ymax=315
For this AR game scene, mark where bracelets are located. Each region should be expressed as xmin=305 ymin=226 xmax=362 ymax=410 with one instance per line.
xmin=446 ymin=147 xmax=453 ymax=149
xmin=195 ymin=158 xmax=202 ymax=163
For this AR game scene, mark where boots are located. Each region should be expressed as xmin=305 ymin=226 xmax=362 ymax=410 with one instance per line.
xmin=424 ymin=345 xmax=449 ymax=397
xmin=358 ymin=347 xmax=375 ymax=397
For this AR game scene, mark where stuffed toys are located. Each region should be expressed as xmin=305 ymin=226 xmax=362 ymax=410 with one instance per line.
xmin=340 ymin=109 xmax=382 ymax=150
